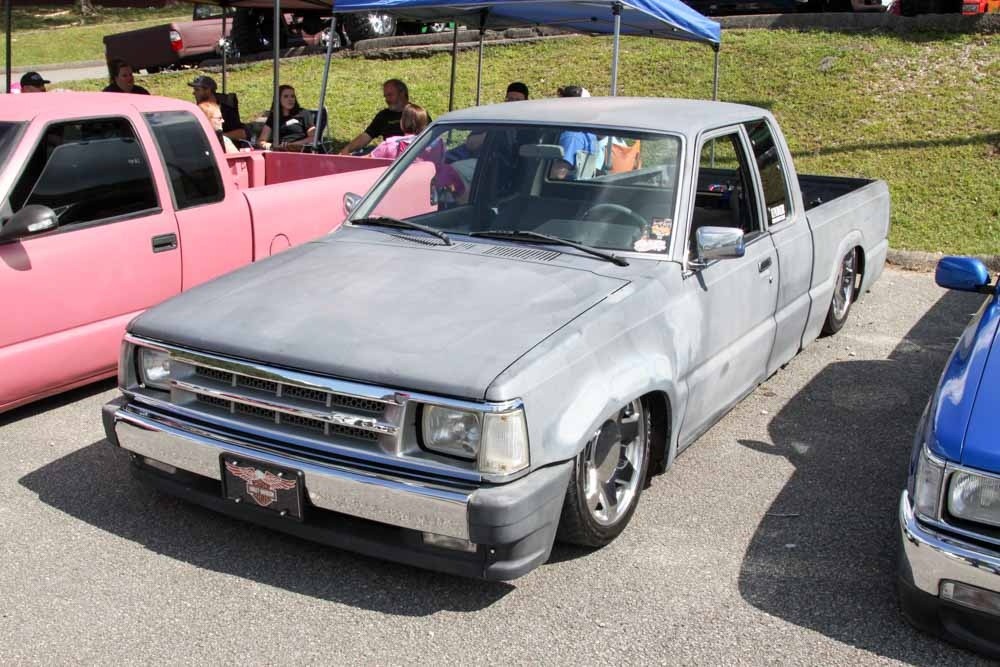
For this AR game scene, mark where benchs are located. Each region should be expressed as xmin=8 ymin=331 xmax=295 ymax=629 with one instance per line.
xmin=492 ymin=196 xmax=732 ymax=256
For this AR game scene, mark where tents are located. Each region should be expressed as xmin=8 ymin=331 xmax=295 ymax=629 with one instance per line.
xmin=313 ymin=0 xmax=720 ymax=167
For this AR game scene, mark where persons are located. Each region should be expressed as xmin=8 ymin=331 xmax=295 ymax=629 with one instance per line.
xmin=441 ymin=80 xmax=618 ymax=204
xmin=255 ymin=85 xmax=315 ymax=152
xmin=102 ymin=56 xmax=151 ymax=95
xmin=337 ymin=79 xmax=433 ymax=155
xmin=362 ymin=102 xmax=464 ymax=195
xmin=187 ymin=76 xmax=246 ymax=138
xmin=20 ymin=71 xmax=50 ymax=93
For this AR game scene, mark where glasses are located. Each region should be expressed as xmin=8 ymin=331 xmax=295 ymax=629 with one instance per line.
xmin=210 ymin=115 xmax=225 ymax=120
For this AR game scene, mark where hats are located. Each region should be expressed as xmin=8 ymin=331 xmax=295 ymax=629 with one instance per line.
xmin=187 ymin=76 xmax=218 ymax=91
xmin=20 ymin=71 xmax=51 ymax=84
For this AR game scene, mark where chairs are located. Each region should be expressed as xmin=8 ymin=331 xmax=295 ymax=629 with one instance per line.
xmin=216 ymin=93 xmax=334 ymax=154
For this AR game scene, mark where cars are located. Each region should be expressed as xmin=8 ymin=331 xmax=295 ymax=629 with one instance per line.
xmin=897 ymin=255 xmax=1000 ymax=662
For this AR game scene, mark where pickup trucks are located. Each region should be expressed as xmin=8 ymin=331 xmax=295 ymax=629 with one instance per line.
xmin=101 ymin=0 xmax=348 ymax=80
xmin=100 ymin=95 xmax=891 ymax=582
xmin=0 ymin=91 xmax=479 ymax=418
